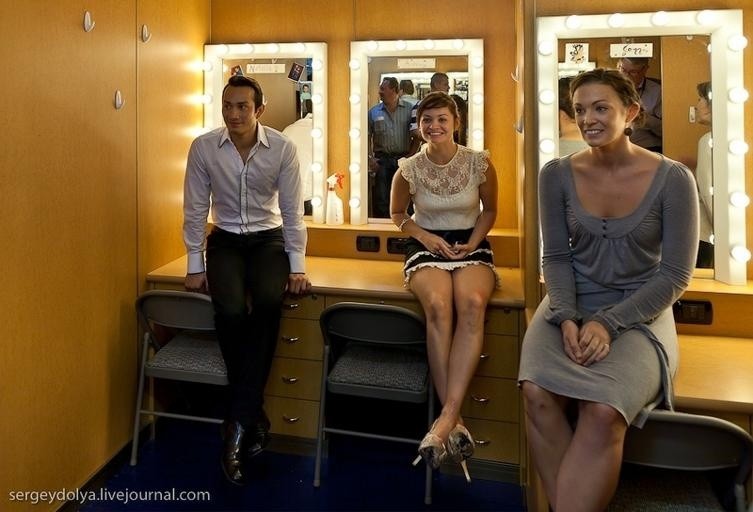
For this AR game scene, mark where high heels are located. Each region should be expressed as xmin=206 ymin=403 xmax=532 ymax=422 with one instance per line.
xmin=413 ymin=418 xmax=448 ymax=468
xmin=448 ymin=424 xmax=476 ymax=482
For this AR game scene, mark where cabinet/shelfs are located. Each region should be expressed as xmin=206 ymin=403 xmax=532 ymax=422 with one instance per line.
xmin=426 ymin=304 xmax=526 ymax=484
xmin=235 ymin=295 xmax=324 ymax=453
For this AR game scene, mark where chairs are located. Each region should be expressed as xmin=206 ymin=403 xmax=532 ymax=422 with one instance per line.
xmin=128 ymin=289 xmax=232 ymax=467
xmin=313 ymin=302 xmax=432 ymax=505
xmin=571 ymin=410 xmax=752 ymax=512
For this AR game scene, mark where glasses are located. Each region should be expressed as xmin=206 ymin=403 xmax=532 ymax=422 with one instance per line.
xmin=618 ymin=64 xmax=646 ymax=77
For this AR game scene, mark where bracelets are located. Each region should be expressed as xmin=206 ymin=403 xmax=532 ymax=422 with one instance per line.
xmin=396 ymin=218 xmax=413 ymax=233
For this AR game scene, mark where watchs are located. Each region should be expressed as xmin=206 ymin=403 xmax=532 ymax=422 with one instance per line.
xmin=368 ymin=153 xmax=372 ymax=160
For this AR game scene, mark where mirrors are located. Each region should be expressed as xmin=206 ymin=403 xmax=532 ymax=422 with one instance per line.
xmin=533 ymin=8 xmax=746 ymax=286
xmin=203 ymin=42 xmax=327 ymax=224
xmin=349 ymin=40 xmax=486 ymax=227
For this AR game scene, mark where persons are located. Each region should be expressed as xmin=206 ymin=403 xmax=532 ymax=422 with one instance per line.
xmin=516 ymin=68 xmax=699 ymax=511
xmin=232 ymin=72 xmax=236 ymax=75
xmin=291 ymin=65 xmax=300 ymax=79
xmin=235 ymin=67 xmax=242 ymax=76
xmin=368 ymin=72 xmax=465 ymax=218
xmin=558 ymin=57 xmax=662 ymax=158
xmin=182 ymin=75 xmax=312 ymax=483
xmin=390 ymin=92 xmax=501 ymax=484
xmin=281 ymin=99 xmax=312 ymax=215
xmin=301 ymin=85 xmax=311 ymax=99
xmin=694 ymin=81 xmax=715 ymax=268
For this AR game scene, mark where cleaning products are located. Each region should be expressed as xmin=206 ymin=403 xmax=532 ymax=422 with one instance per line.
xmin=326 ymin=172 xmax=345 ymax=226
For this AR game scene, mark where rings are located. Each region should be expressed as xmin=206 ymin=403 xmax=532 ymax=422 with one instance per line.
xmin=604 ymin=342 xmax=611 ymax=348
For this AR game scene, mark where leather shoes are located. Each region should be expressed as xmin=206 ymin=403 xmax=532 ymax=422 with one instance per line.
xmin=244 ymin=417 xmax=271 ymax=458
xmin=219 ymin=416 xmax=247 ymax=484
